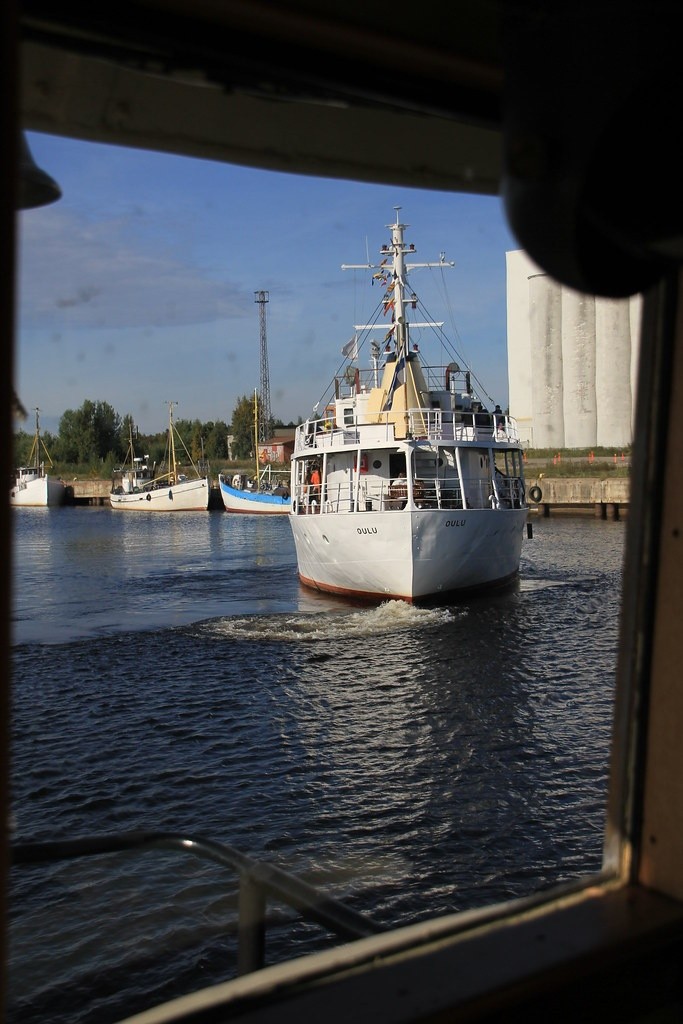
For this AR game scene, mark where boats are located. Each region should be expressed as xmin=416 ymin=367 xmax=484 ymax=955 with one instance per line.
xmin=109 ymin=400 xmax=211 ymax=512
xmin=217 ymin=388 xmax=291 ymax=514
xmin=8 ymin=408 xmax=66 ymax=506
xmin=287 ymin=206 xmax=531 ymax=607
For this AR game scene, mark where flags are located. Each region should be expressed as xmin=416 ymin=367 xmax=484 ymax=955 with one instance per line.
xmin=341 ymin=338 xmax=356 ymax=360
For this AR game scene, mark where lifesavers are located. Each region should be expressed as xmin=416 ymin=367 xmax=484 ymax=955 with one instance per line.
xmin=529 ymin=486 xmax=542 ymax=502
xmin=65 ymin=486 xmax=74 ymax=498
xmin=233 ymin=479 xmax=239 ymax=490
xmin=282 ymin=493 xmax=288 ymax=500
xmin=146 ymin=494 xmax=151 ymax=501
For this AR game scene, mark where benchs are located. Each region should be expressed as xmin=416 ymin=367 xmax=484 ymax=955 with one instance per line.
xmin=386 ymin=484 xmax=425 ymax=509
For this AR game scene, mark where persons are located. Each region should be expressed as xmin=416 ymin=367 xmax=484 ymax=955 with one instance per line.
xmin=492 ymin=405 xmax=503 ymax=425
xmin=391 ymin=473 xmax=406 ymax=510
xmin=304 ymin=467 xmax=321 ymax=504
xmin=414 ymin=481 xmax=423 ymax=509
